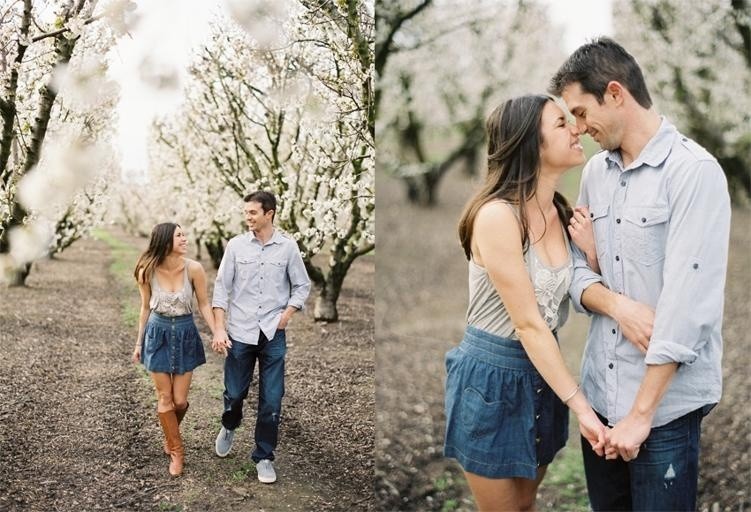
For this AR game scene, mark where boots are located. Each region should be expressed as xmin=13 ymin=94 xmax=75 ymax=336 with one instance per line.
xmin=157 ymin=402 xmax=189 ymax=477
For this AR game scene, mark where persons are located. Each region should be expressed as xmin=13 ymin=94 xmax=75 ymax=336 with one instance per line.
xmin=547 ymin=36 xmax=732 ymax=512
xmin=442 ymin=94 xmax=600 ymax=512
xmin=132 ymin=223 xmax=233 ymax=478
xmin=211 ymin=190 xmax=311 ymax=484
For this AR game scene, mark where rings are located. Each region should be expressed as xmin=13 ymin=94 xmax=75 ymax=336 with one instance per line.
xmin=572 ymin=221 xmax=577 ymax=228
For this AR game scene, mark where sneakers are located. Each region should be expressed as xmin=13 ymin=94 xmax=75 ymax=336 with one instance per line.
xmin=256 ymin=459 xmax=277 ymax=484
xmin=216 ymin=425 xmax=235 ymax=458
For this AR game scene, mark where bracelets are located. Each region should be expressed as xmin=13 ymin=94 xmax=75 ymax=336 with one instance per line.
xmin=135 ymin=344 xmax=143 ymax=346
xmin=561 ymin=385 xmax=580 ymax=404
